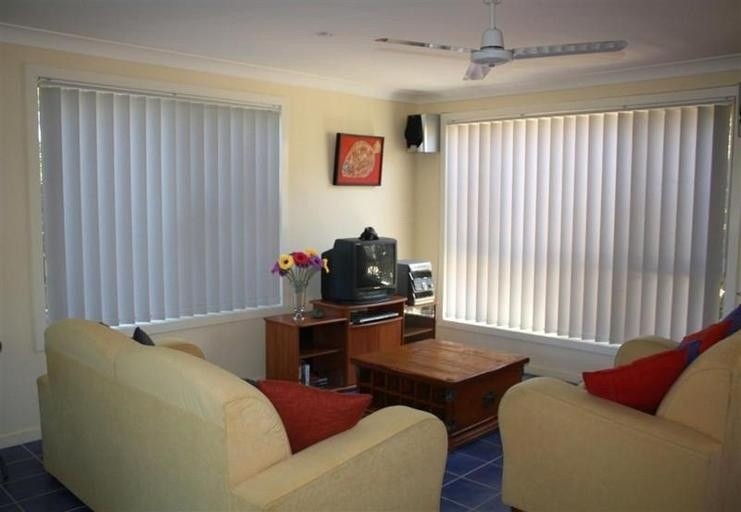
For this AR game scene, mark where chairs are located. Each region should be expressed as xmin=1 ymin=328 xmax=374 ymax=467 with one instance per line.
xmin=497 ymin=328 xmax=741 ymax=512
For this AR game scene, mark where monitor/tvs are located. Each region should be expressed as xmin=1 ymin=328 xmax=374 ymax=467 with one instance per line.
xmin=318 ymin=236 xmax=400 ymax=308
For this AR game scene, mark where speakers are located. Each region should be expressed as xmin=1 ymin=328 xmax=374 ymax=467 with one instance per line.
xmin=404 ymin=112 xmax=440 ymax=152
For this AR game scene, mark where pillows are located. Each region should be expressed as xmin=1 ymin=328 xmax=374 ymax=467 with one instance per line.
xmin=681 ymin=320 xmax=736 ymax=355
xmin=582 ymin=346 xmax=689 ymax=416
xmin=679 ymin=340 xmax=701 ymax=365
xmin=721 ymin=303 xmax=741 ymax=336
xmin=256 ymin=379 xmax=374 ymax=455
xmin=132 ymin=326 xmax=155 ymax=346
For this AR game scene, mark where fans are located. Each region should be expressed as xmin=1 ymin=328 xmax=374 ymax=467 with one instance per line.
xmin=374 ymin=0 xmax=628 ymax=81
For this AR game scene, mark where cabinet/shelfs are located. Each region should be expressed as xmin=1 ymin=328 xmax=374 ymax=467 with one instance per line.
xmin=401 ymin=301 xmax=437 ymax=343
xmin=263 ymin=295 xmax=408 ymax=392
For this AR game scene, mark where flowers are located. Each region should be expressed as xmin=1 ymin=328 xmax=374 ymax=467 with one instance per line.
xmin=270 ymin=246 xmax=330 ymax=294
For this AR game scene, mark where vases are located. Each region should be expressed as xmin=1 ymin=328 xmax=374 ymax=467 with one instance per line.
xmin=290 ymin=280 xmax=308 ymax=321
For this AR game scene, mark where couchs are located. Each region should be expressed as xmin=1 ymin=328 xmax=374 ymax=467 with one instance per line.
xmin=36 ymin=318 xmax=449 ymax=512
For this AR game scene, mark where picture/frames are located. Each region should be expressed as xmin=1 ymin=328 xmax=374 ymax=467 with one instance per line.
xmin=332 ymin=132 xmax=385 ymax=186
